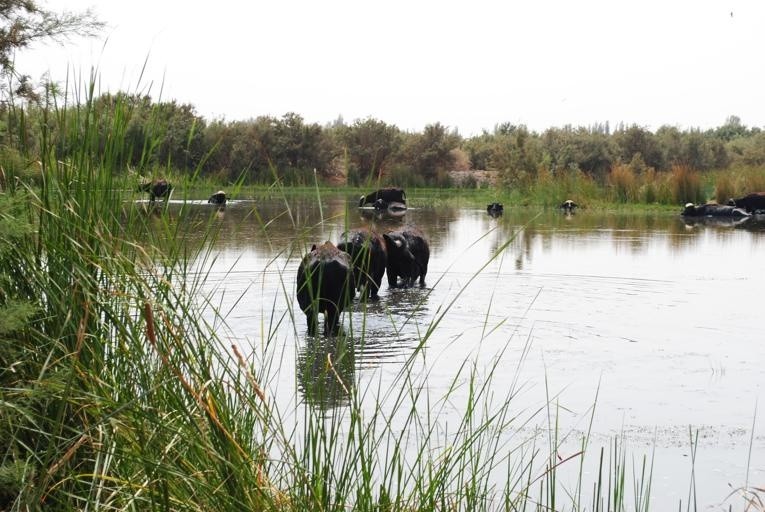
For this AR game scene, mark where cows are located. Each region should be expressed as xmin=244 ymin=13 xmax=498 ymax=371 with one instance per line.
xmin=486 ymin=203 xmax=504 ymax=213
xmin=207 ymin=191 xmax=227 ymax=204
xmin=335 ymin=227 xmax=386 ymax=298
xmin=680 ymin=192 xmax=765 ymax=216
xmin=382 ymin=224 xmax=429 ymax=287
xmin=297 ymin=241 xmax=356 ymax=338
xmin=135 ymin=179 xmax=173 ymax=201
xmin=561 ymin=200 xmax=577 ymax=211
xmin=359 ymin=187 xmax=407 ymax=210
